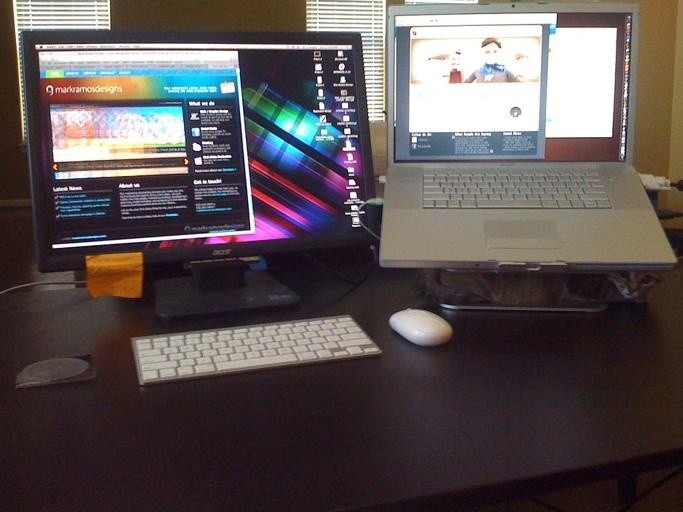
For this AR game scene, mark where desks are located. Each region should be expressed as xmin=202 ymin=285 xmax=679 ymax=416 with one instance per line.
xmin=0 ymin=265 xmax=683 ymax=512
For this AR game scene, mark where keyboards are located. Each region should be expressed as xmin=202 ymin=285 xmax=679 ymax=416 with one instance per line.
xmin=129 ymin=315 xmax=385 ymax=388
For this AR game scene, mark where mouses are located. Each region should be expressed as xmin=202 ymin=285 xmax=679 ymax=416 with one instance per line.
xmin=387 ymin=309 xmax=454 ymax=346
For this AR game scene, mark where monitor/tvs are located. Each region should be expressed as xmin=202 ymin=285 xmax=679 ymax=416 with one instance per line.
xmin=19 ymin=30 xmax=381 ymax=320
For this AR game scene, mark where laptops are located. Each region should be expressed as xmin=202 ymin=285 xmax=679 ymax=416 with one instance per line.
xmin=379 ymin=2 xmax=678 ymax=270
